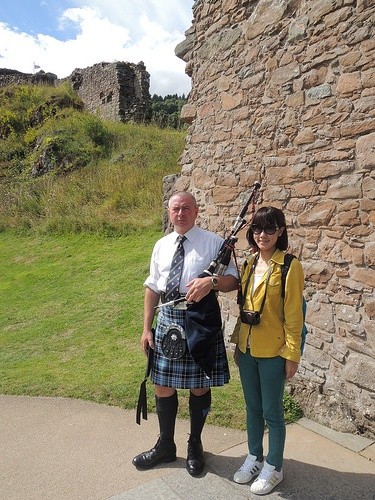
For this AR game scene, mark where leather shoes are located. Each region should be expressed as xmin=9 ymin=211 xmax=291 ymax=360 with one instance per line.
xmin=131 ymin=437 xmax=177 ymax=468
xmin=186 ymin=437 xmax=204 ymax=477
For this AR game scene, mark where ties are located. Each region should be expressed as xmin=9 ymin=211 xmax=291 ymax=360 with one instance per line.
xmin=164 ymin=235 xmax=188 ymax=309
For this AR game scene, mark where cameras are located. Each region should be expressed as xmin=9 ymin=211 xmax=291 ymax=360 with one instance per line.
xmin=241 ymin=310 xmax=260 ymax=326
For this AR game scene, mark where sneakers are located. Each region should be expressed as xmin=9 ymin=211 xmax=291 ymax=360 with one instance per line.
xmin=234 ymin=454 xmax=264 ymax=483
xmin=249 ymin=461 xmax=284 ymax=495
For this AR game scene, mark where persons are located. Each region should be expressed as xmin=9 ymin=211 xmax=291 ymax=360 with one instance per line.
xmin=230 ymin=207 xmax=304 ymax=494
xmin=132 ymin=192 xmax=239 ymax=476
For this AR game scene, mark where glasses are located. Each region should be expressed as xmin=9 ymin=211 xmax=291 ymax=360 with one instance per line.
xmin=251 ymin=224 xmax=280 ymax=235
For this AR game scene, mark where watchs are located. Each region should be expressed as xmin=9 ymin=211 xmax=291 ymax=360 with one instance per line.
xmin=210 ymin=276 xmax=218 ymax=290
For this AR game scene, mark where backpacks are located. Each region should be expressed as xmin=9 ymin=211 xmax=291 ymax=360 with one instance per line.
xmin=243 ymin=253 xmax=308 ymax=356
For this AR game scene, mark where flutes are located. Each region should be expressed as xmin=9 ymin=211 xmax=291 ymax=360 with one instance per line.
xmin=196 ymin=181 xmax=261 ymax=298
